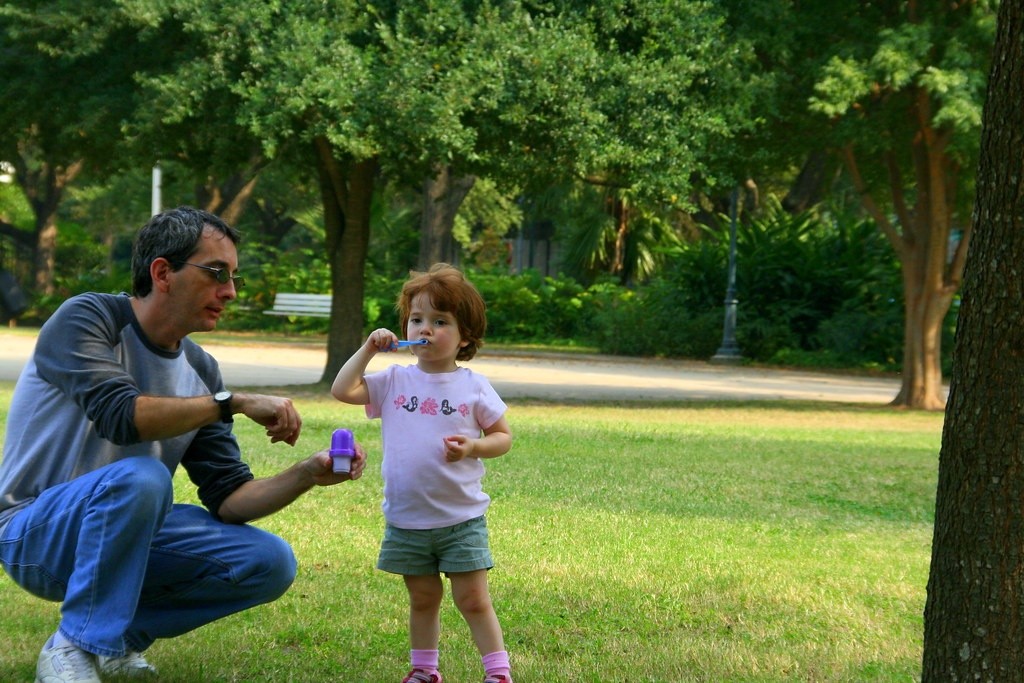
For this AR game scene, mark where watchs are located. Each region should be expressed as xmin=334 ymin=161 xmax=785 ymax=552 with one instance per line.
xmin=213 ymin=388 xmax=233 ymax=423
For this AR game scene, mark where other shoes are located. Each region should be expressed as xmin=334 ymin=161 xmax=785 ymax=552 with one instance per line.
xmin=403 ymin=668 xmax=443 ymax=683
xmin=483 ymin=673 xmax=513 ymax=683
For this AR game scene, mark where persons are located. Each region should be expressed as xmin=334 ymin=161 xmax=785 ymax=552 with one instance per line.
xmin=1 ymin=205 xmax=368 ymax=682
xmin=331 ymin=258 xmax=512 ymax=683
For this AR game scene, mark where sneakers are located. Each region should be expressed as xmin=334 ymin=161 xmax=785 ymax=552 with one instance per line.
xmin=98 ymin=646 xmax=158 ymax=676
xmin=33 ymin=630 xmax=101 ymax=683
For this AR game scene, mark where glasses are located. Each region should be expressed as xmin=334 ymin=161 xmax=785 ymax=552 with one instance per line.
xmin=172 ymin=258 xmax=246 ymax=291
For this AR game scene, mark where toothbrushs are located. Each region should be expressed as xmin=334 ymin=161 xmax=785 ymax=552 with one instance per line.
xmin=382 ymin=340 xmax=428 ymax=352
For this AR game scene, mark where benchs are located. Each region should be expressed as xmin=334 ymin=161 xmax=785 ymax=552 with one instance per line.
xmin=261 ymin=292 xmax=333 ymax=338
